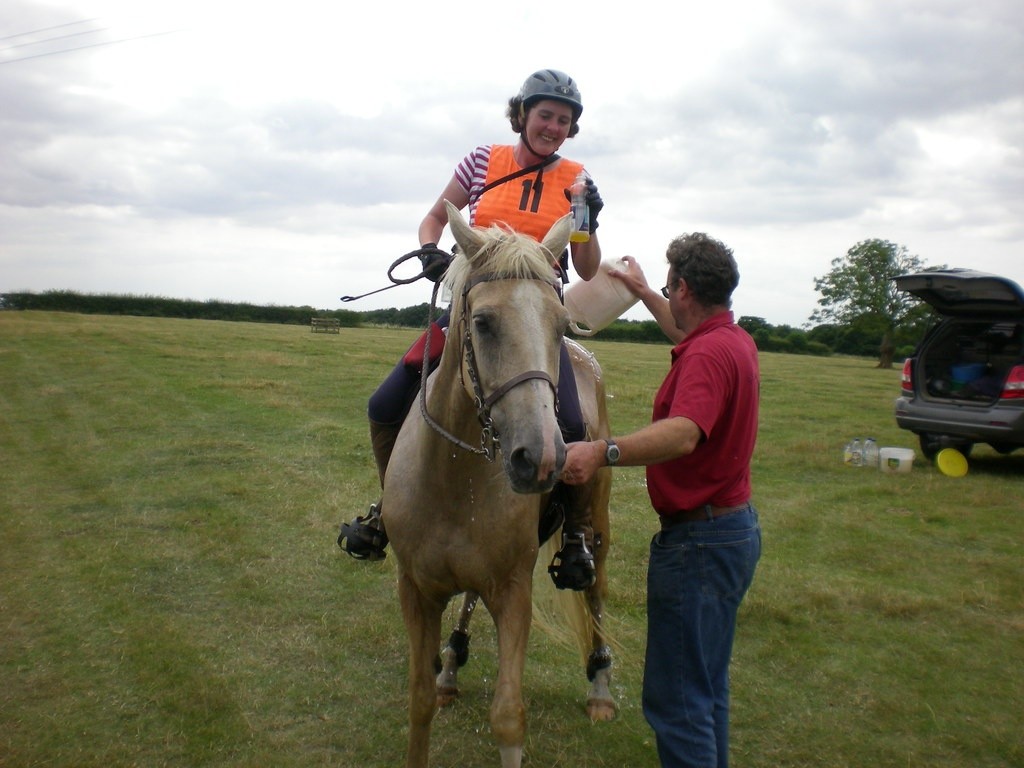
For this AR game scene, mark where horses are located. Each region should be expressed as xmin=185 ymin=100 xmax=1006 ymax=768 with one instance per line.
xmin=380 ymin=198 xmax=619 ymax=768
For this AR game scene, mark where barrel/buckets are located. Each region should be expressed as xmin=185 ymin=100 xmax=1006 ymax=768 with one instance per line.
xmin=880 ymin=448 xmax=916 ymax=474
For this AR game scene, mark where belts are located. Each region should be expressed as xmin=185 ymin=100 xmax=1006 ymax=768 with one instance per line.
xmin=659 ymin=502 xmax=749 ymax=527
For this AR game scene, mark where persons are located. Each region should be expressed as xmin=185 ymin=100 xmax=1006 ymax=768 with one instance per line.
xmin=346 ymin=69 xmax=604 ymax=591
xmin=560 ymin=232 xmax=762 ymax=768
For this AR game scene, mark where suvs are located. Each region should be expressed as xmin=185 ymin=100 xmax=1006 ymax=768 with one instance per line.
xmin=886 ymin=268 xmax=1024 ymax=464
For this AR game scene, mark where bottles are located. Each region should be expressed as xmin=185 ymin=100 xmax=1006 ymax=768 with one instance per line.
xmin=863 ymin=438 xmax=871 ymax=466
xmin=844 ymin=447 xmax=853 ymax=467
xmin=569 ymin=171 xmax=592 ymax=243
xmin=563 ymin=258 xmax=642 ymax=337
xmin=853 ymin=438 xmax=863 ymax=467
xmin=867 ymin=440 xmax=879 ymax=467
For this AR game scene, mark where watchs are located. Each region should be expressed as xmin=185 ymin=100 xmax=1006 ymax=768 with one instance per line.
xmin=603 ymin=438 xmax=621 ymax=467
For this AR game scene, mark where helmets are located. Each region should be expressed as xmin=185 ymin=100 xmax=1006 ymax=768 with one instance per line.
xmin=520 ymin=69 xmax=583 ymax=124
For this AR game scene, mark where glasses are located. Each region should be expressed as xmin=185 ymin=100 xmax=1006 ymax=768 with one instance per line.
xmin=661 ymin=278 xmax=692 ymax=299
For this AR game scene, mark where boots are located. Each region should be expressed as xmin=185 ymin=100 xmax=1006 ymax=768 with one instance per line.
xmin=349 ymin=409 xmax=405 ymax=554
xmin=561 ymin=423 xmax=595 ymax=584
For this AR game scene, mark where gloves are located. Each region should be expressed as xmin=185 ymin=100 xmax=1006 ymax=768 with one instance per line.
xmin=564 ymin=178 xmax=604 ymax=234
xmin=418 ymin=243 xmax=453 ymax=282
